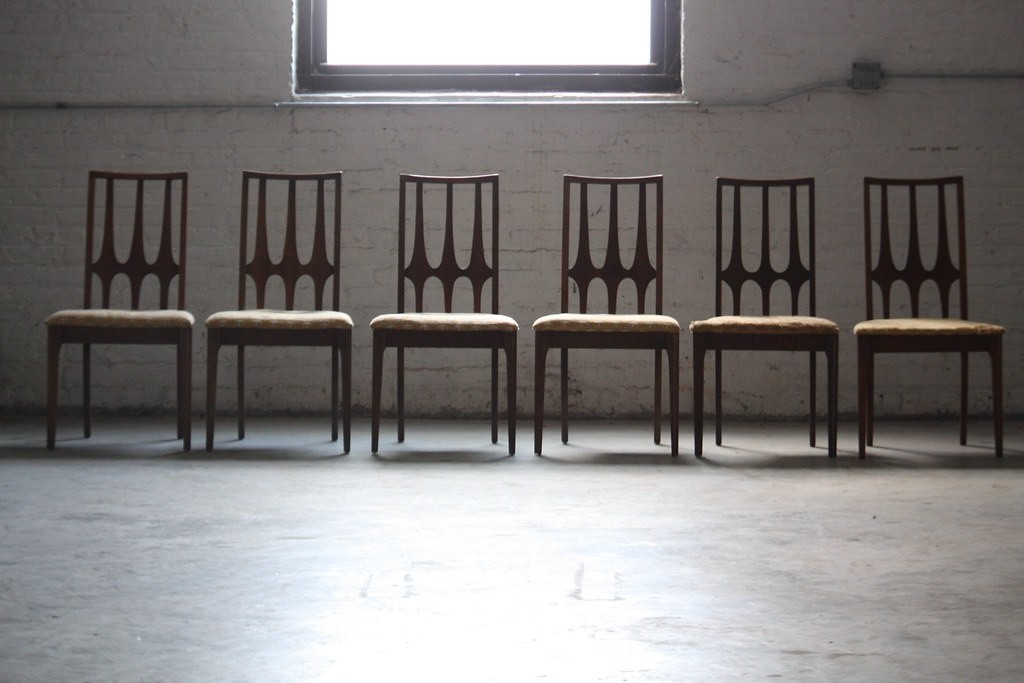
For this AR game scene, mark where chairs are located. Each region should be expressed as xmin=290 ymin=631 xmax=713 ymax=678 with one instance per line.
xmin=687 ymin=177 xmax=842 ymax=461
xmin=44 ymin=167 xmax=198 ymax=456
xmin=203 ymin=170 xmax=357 ymax=456
xmin=857 ymin=160 xmax=1008 ymax=465
xmin=531 ymin=172 xmax=682 ymax=455
xmin=369 ymin=172 xmax=521 ymax=458
